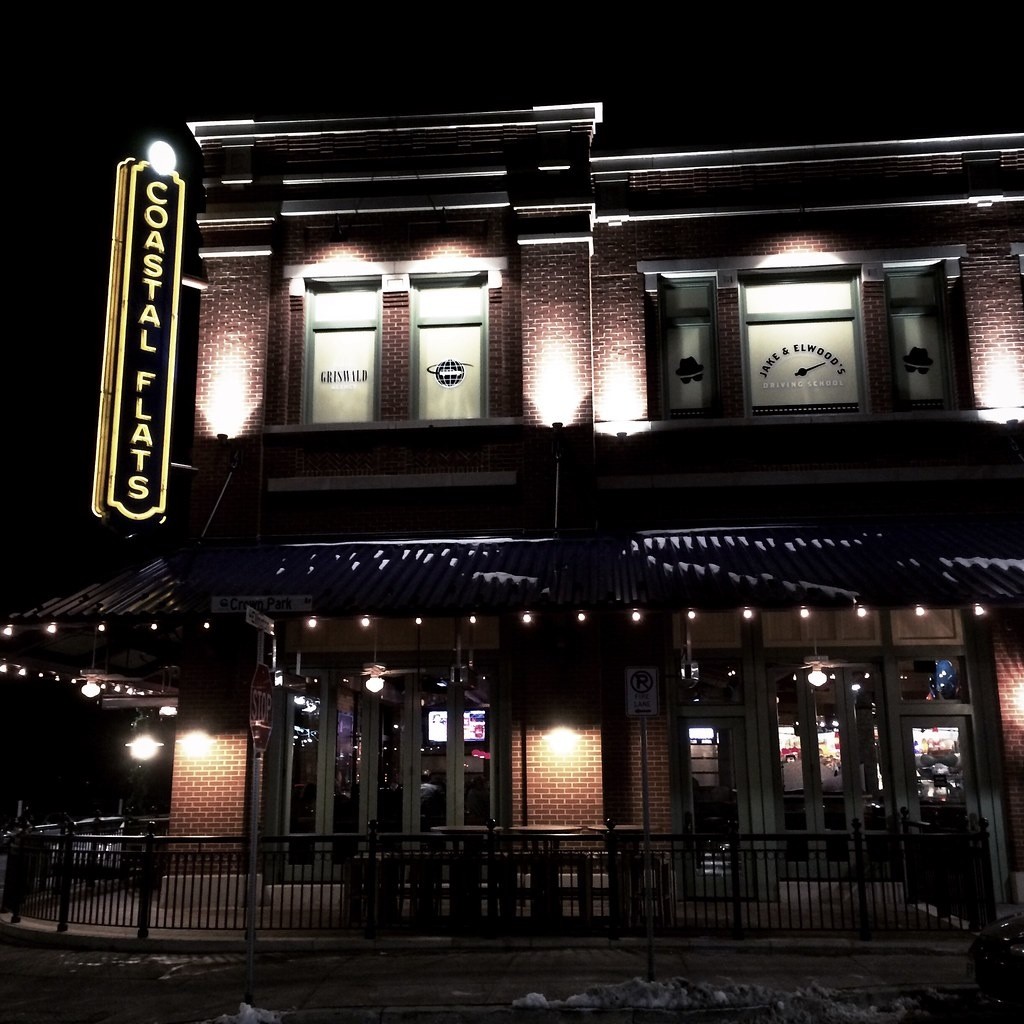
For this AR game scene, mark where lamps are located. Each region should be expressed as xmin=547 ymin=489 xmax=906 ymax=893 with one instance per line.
xmin=125 ymin=717 xmax=164 ymax=760
xmin=284 ymin=654 xmax=311 ymax=688
xmin=82 ymin=623 xmax=101 ymax=698
xmin=552 ymin=422 xmax=563 ymax=433
xmin=804 ymin=634 xmax=827 ymax=686
xmin=678 ymin=623 xmax=701 ymax=683
xmin=217 ymin=434 xmax=228 ymax=444
xmin=450 ymin=617 xmax=464 ymax=684
xmin=366 ymin=617 xmax=385 ymax=693
xmin=617 ymin=433 xmax=627 ymax=444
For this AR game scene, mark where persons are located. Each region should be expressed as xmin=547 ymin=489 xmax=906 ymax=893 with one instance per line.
xmin=334 ymin=781 xmax=354 ymax=820
xmin=421 ymin=779 xmax=491 ymax=819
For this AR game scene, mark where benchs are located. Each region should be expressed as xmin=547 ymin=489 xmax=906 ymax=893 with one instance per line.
xmin=352 ymin=852 xmax=675 ymax=937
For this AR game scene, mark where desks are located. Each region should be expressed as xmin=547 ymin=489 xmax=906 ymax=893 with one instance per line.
xmin=431 ymin=823 xmax=503 ymax=937
xmin=589 ymin=823 xmax=657 ymax=937
xmin=510 ymin=824 xmax=582 ymax=937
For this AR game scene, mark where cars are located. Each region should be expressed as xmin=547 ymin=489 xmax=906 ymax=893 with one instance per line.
xmin=52 ymin=816 xmax=161 ymax=880
xmin=2 ymin=823 xmax=61 ymax=850
xmin=700 ymin=840 xmax=733 ymax=875
xmin=967 ymin=909 xmax=1023 ymax=1001
